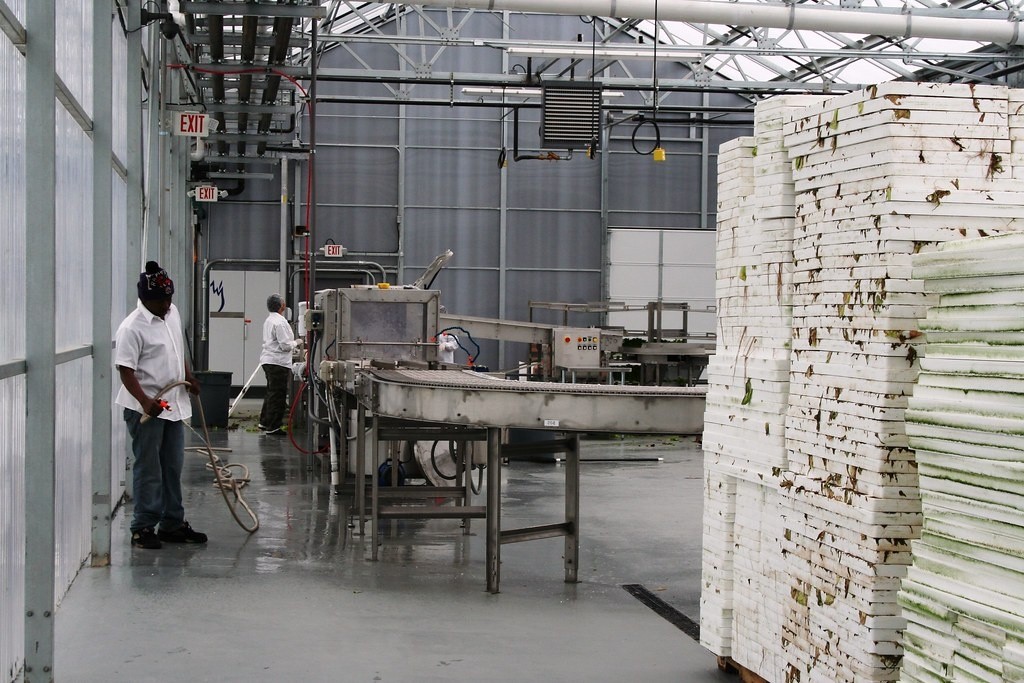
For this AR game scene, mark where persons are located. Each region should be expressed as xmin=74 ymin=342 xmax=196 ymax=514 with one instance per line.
xmin=114 ymin=261 xmax=208 ymax=549
xmin=439 ymin=304 xmax=459 ymax=364
xmin=258 ymin=294 xmax=303 ymax=435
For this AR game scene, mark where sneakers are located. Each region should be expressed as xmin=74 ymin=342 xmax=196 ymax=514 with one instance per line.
xmin=131 ymin=526 xmax=162 ymax=549
xmin=157 ymin=523 xmax=208 ymax=544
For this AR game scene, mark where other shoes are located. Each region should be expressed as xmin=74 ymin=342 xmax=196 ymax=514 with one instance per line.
xmin=258 ymin=422 xmax=269 ymax=431
xmin=266 ymin=427 xmax=287 ymax=436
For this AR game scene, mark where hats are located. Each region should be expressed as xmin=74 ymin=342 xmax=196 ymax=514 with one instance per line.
xmin=137 ymin=260 xmax=174 ymax=300
